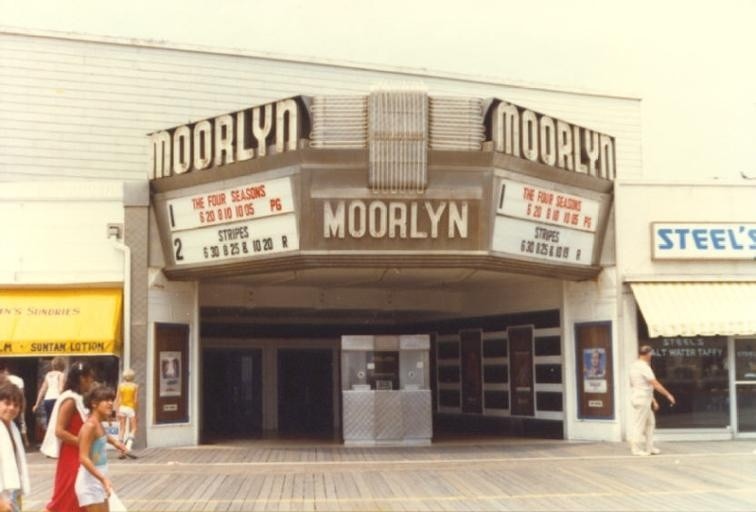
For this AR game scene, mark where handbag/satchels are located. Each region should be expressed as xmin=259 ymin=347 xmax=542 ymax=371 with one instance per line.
xmin=108 ymin=488 xmax=127 ymax=512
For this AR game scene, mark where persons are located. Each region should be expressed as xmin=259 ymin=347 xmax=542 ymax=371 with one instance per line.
xmin=0 ymin=356 xmax=139 ymax=512
xmin=627 ymin=343 xmax=677 ymax=458
xmin=585 ymin=349 xmax=604 ymax=380
